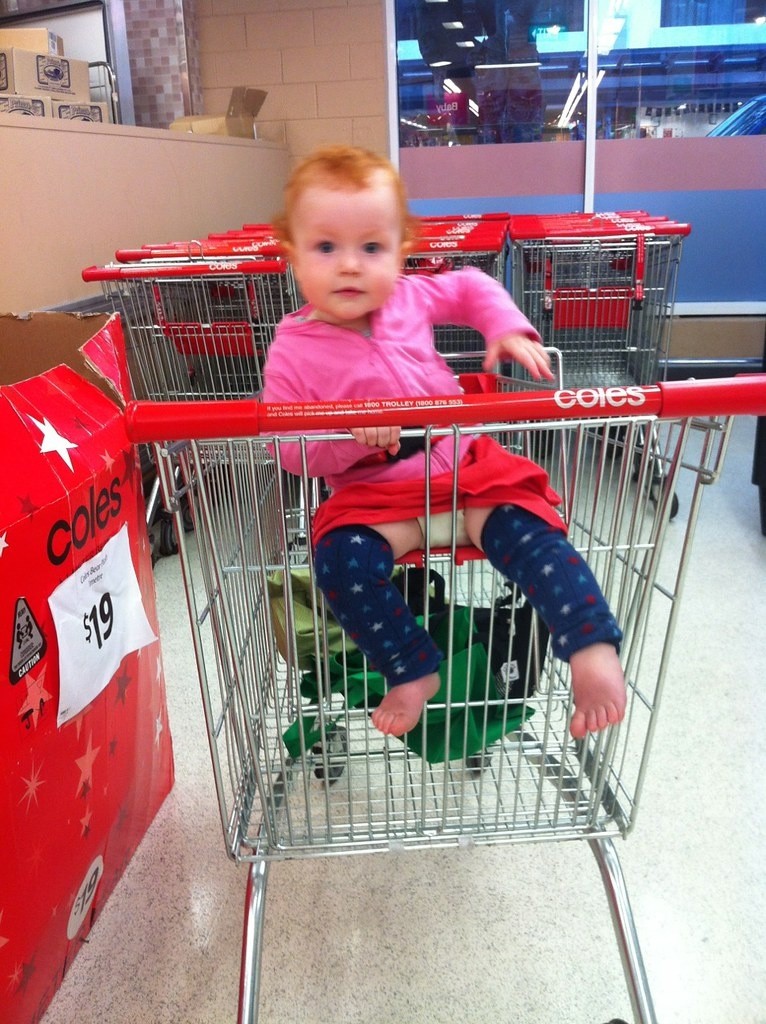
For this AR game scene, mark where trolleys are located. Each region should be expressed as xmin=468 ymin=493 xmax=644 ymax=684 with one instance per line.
xmin=81 ymin=207 xmax=691 ymax=557
xmin=121 ymin=346 xmax=766 ymax=1024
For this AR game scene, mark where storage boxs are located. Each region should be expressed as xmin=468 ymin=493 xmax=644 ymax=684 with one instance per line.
xmin=0 ymin=28 xmax=110 ymax=123
xmin=168 ymin=87 xmax=268 ymax=139
xmin=0 ymin=309 xmax=177 ymax=1024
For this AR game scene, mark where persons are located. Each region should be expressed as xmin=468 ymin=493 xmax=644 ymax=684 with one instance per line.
xmin=263 ymin=147 xmax=627 ymax=737
xmin=417 ymin=0 xmax=544 ymax=144
